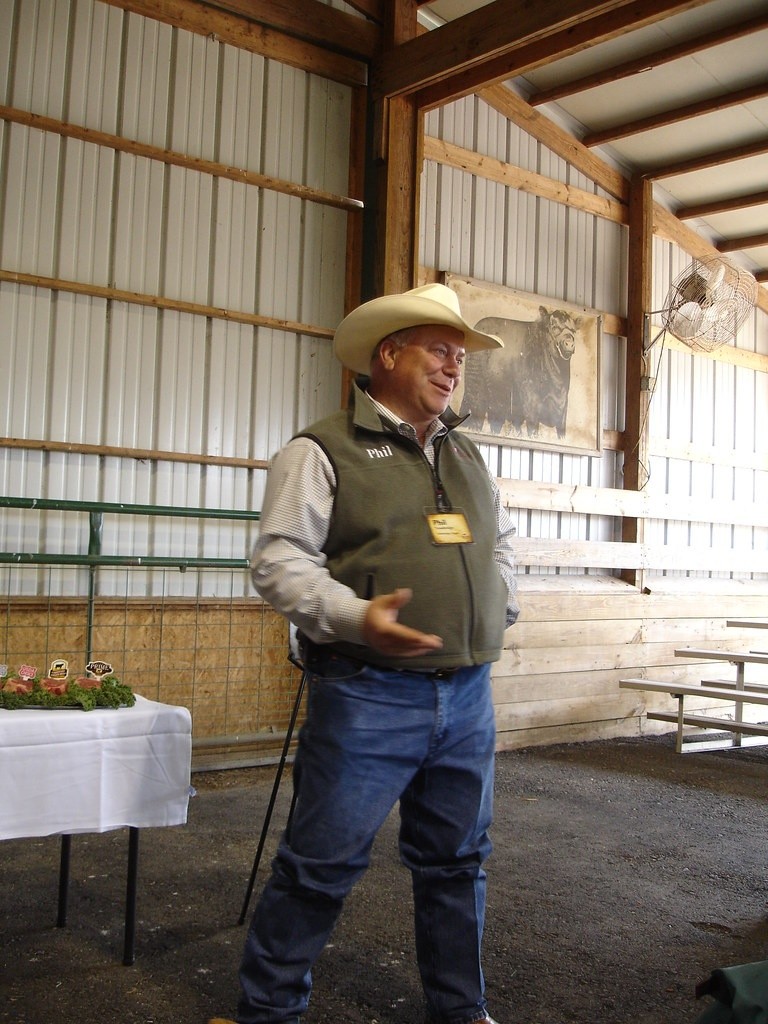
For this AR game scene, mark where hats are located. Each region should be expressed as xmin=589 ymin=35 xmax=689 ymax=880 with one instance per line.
xmin=335 ymin=284 xmax=505 ymax=376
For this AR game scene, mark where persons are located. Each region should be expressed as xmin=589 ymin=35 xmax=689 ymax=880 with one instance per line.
xmin=239 ymin=283 xmax=519 ymax=1024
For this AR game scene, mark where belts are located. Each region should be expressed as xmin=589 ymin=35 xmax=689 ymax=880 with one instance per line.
xmin=345 ymin=645 xmax=463 ymax=681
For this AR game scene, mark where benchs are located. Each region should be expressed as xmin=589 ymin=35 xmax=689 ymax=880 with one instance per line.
xmin=647 ymin=711 xmax=768 ymax=737
xmin=701 ymin=678 xmax=768 ymax=693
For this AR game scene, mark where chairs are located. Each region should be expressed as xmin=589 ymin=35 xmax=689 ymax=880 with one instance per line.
xmin=236 ymin=616 xmax=313 ymax=926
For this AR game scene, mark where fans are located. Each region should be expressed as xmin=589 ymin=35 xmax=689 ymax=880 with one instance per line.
xmin=641 ymin=250 xmax=758 ymax=354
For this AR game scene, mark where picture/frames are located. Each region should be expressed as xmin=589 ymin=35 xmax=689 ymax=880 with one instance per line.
xmin=440 ymin=271 xmax=602 ymax=458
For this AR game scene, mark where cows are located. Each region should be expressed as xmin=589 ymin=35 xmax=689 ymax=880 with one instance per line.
xmin=459 ymin=305 xmax=583 ymax=440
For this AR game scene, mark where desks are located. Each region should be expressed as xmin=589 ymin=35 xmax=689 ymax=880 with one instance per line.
xmin=674 ymin=649 xmax=768 ymax=746
xmin=749 ymin=650 xmax=768 ymax=654
xmin=620 ymin=679 xmax=768 ymax=754
xmin=727 ymin=621 xmax=768 ymax=629
xmin=0 ymin=686 xmax=191 ymax=967
